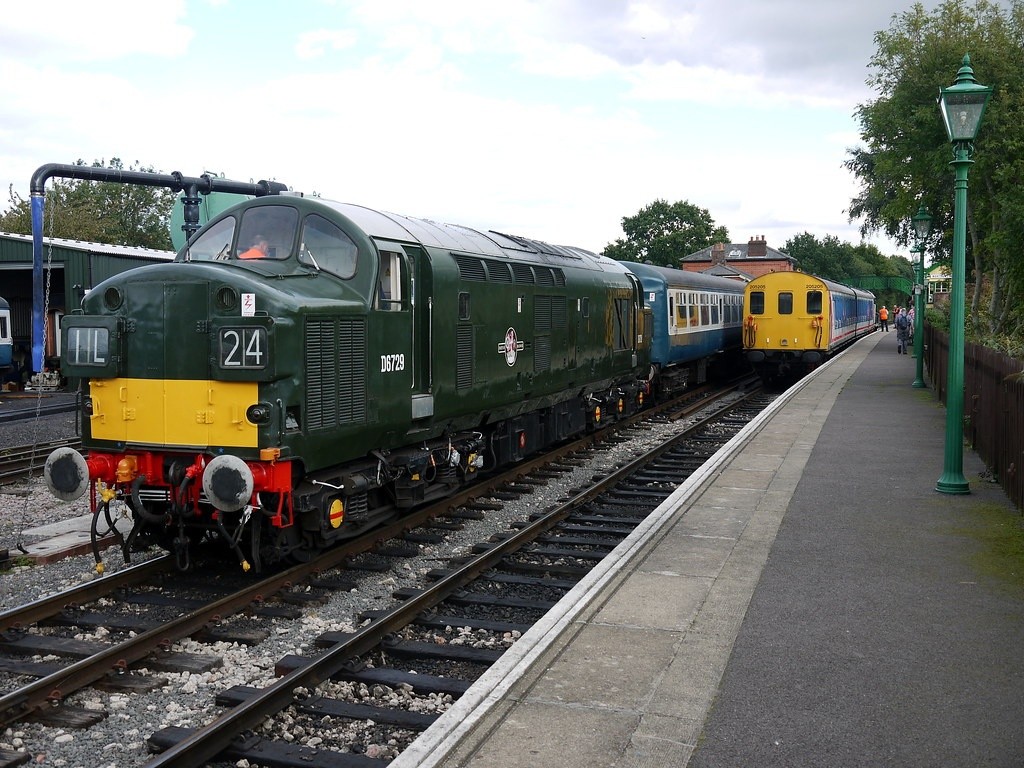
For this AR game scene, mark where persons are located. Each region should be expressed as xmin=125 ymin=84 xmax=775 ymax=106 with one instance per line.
xmin=894 ymin=307 xmax=911 ymax=355
xmin=879 ymin=305 xmax=889 ymax=332
xmin=237 ymin=235 xmax=270 ymax=258
xmin=891 ymin=300 xmax=915 ymax=346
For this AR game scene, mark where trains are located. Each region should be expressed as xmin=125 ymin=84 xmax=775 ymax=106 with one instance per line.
xmin=58 ymin=190 xmax=746 ymax=589
xmin=740 ymin=269 xmax=881 ymax=377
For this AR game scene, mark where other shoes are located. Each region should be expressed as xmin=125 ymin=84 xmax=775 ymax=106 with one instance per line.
xmin=903 ymin=350 xmax=907 ymax=354
xmin=898 ymin=345 xmax=901 ymax=354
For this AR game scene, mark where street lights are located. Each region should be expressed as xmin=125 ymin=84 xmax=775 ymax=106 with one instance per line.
xmin=909 ymin=200 xmax=928 ymax=389
xmin=931 ymin=51 xmax=996 ymax=496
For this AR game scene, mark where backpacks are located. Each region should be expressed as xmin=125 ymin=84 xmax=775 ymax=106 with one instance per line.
xmin=898 ymin=314 xmax=908 ymax=330
